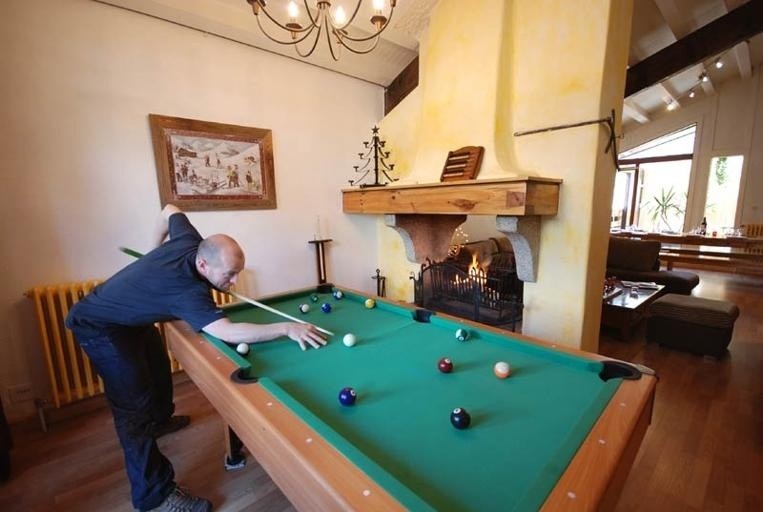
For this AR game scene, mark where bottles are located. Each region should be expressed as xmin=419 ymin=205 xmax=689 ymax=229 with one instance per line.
xmin=700 ymin=216 xmax=708 ymax=235
xmin=603 ymin=276 xmax=616 ymax=295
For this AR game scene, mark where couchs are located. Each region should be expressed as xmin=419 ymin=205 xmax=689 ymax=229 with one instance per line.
xmin=456 ymin=236 xmax=515 ymax=281
xmin=608 ymin=236 xmax=700 ymax=296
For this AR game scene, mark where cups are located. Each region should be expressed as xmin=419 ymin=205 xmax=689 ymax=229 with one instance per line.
xmin=720 ymin=226 xmax=744 ymax=237
xmin=630 ymin=286 xmax=638 ymax=297
xmin=713 ymin=231 xmax=717 ymax=236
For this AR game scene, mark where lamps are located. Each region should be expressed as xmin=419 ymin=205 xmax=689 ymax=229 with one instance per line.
xmin=244 ymin=0 xmax=399 ymax=64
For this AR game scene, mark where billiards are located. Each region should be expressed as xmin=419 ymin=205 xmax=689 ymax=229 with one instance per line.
xmin=235 ymin=291 xmax=513 ymax=428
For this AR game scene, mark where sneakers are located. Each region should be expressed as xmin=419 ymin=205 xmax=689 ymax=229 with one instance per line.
xmin=152 ymin=416 xmax=190 ymax=438
xmin=147 ymin=488 xmax=212 ymax=512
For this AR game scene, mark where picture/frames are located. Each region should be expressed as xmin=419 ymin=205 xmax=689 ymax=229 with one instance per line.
xmin=148 ymin=112 xmax=277 ymax=211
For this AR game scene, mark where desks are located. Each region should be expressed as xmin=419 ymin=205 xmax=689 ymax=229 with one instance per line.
xmin=155 ymin=280 xmax=659 ymax=512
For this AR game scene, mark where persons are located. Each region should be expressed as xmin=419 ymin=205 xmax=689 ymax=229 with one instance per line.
xmin=175 ymin=152 xmax=253 ymax=192
xmin=64 ymin=204 xmax=328 ymax=512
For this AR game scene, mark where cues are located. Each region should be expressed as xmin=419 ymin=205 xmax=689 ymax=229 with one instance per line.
xmin=114 ymin=244 xmax=337 ymax=336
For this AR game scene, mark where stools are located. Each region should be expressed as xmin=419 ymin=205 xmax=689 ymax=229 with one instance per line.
xmin=650 ymin=294 xmax=740 ymax=351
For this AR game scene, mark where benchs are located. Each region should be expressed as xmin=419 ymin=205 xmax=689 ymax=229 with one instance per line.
xmin=409 ymin=257 xmax=519 ymax=331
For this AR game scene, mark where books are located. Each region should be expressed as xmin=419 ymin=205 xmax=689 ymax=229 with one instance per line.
xmin=621 ymin=280 xmax=658 ymax=290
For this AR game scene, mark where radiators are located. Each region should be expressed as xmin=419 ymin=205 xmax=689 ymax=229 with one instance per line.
xmin=23 ymin=281 xmax=242 ymax=408
